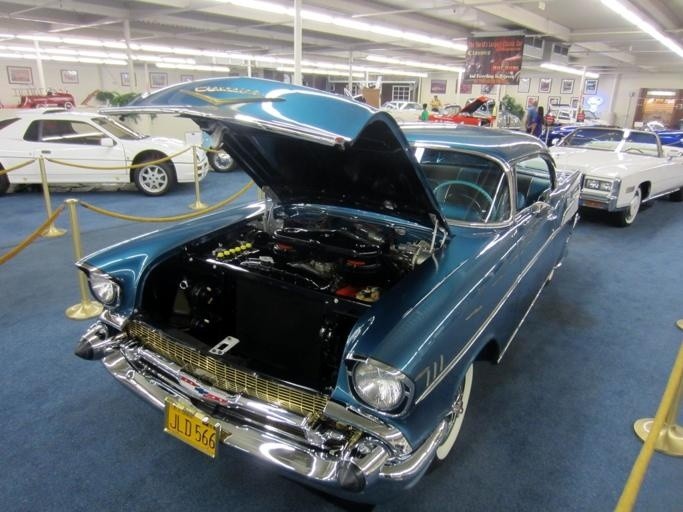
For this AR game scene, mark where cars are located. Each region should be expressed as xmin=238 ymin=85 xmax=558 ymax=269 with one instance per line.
xmin=75 ymin=77 xmax=587 ymax=506
xmin=515 ymin=126 xmax=683 ymax=229
xmin=538 ymin=126 xmax=682 ymax=149
xmin=379 ymin=96 xmax=496 ymax=129
xmin=1 ymin=110 xmax=236 ymax=196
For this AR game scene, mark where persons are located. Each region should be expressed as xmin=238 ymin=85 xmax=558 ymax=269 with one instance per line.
xmin=419 ymin=94 xmax=443 ymax=122
xmin=525 ymin=97 xmax=543 ymax=137
